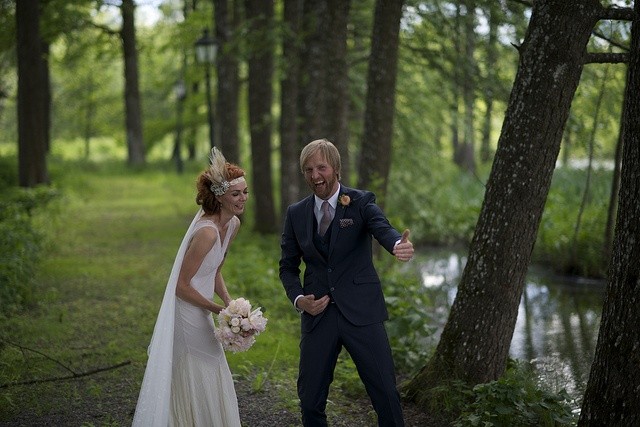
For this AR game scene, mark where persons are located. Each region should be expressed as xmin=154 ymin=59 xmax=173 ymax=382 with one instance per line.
xmin=278 ymin=139 xmax=415 ymax=427
xmin=131 ymin=146 xmax=250 ymax=427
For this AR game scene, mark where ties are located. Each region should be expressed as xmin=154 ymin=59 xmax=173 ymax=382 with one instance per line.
xmin=319 ymin=202 xmax=332 ymax=235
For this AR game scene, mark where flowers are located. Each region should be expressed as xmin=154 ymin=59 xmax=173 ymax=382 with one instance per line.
xmin=213 ymin=297 xmax=268 ymax=353
